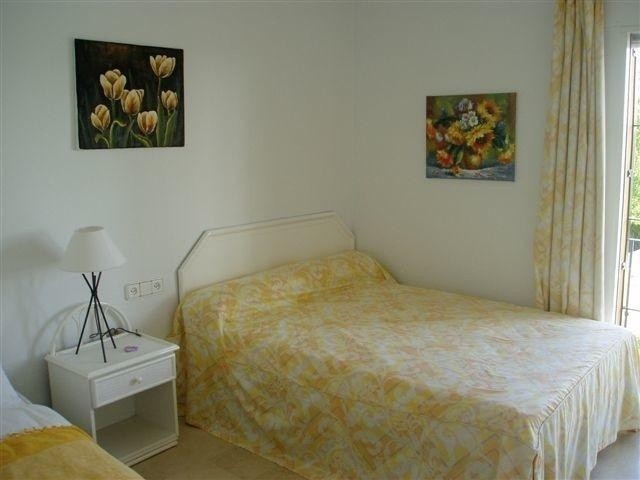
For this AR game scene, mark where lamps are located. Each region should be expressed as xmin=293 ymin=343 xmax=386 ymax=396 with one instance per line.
xmin=59 ymin=225 xmax=126 ymax=363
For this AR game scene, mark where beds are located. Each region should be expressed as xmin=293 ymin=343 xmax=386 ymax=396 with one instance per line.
xmin=163 ymin=211 xmax=640 ymax=480
xmin=0 ymin=366 xmax=146 ymax=480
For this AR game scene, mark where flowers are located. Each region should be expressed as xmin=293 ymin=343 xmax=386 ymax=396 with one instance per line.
xmin=426 ymin=98 xmax=514 ymax=176
xmin=90 ymin=55 xmax=183 ymax=149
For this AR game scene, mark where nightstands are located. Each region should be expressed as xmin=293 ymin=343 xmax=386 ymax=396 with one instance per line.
xmin=43 ymin=302 xmax=181 ymax=467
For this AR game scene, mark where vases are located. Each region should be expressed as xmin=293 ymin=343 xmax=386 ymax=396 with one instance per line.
xmin=458 ymin=147 xmax=497 ymax=170
